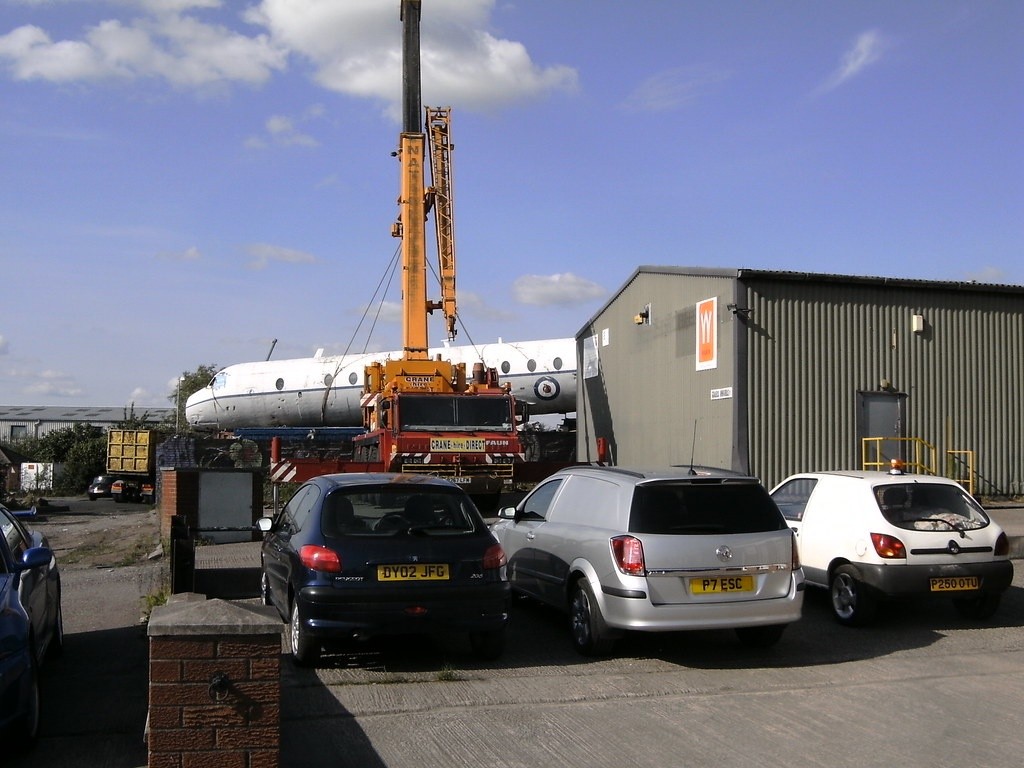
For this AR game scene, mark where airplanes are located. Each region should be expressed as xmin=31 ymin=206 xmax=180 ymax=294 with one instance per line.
xmin=183 ymin=336 xmax=578 ymax=433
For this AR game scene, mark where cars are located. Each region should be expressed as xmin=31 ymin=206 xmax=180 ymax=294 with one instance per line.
xmin=0 ymin=500 xmax=63 ymax=760
xmin=763 ymin=457 xmax=1014 ymax=628
xmin=483 ymin=462 xmax=807 ymax=655
xmin=253 ymin=472 xmax=512 ymax=667
xmin=88 ymin=475 xmax=117 ymax=501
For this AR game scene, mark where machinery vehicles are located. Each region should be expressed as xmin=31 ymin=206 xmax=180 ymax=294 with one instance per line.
xmin=350 ymin=1 xmax=530 ymax=504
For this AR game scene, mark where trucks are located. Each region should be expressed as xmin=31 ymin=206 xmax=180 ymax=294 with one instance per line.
xmin=104 ymin=427 xmax=166 ymax=504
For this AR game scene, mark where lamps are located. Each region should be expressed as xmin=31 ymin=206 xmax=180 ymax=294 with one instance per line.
xmin=634 ymin=312 xmax=649 ymax=325
xmin=727 ymin=303 xmax=754 ymax=322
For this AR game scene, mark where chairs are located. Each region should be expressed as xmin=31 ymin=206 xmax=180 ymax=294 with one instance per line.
xmin=883 ymin=487 xmax=908 ymax=512
xmin=403 ymin=496 xmax=438 ymax=528
xmin=336 ymin=498 xmax=355 ymax=530
